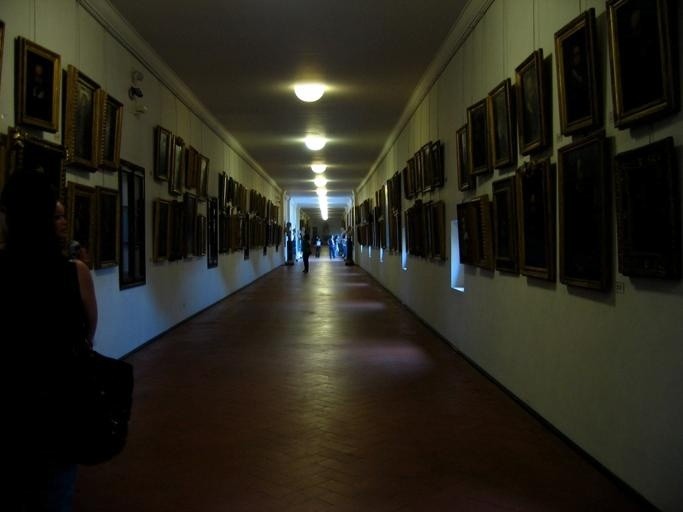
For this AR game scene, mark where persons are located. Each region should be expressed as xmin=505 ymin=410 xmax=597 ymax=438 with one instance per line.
xmin=1 ymin=150 xmax=134 ymax=510
xmin=299 ymin=227 xmax=347 ymax=273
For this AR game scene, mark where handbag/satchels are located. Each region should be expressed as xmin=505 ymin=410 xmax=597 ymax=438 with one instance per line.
xmin=72 ymin=343 xmax=132 ymax=466
